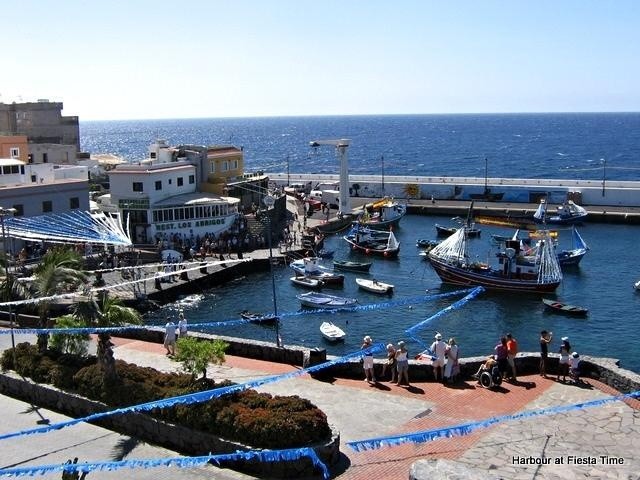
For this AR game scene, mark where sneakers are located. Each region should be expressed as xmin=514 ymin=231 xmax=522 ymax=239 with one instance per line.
xmin=389 ymin=379 xmax=410 ymax=387
xmin=372 ymin=378 xmax=376 ymax=382
xmin=364 ymin=377 xmax=369 ymax=382
xmin=502 ymin=377 xmax=517 ymax=384
xmin=540 ymin=373 xmax=582 ymax=384
xmin=166 ymin=352 xmax=175 ymax=356
xmin=433 ymin=377 xmax=457 ymax=383
xmin=472 ymin=374 xmax=479 ymax=378
xmin=378 ymin=374 xmax=385 ymax=377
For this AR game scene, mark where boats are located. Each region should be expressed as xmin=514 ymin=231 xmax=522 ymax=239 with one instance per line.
xmin=237 ymin=309 xmax=280 ymax=326
xmin=468 ymin=192 xmax=505 ymax=201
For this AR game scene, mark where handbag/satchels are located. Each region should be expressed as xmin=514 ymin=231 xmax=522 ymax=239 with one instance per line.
xmin=452 ymin=360 xmax=460 ymax=376
xmin=364 ymin=336 xmax=372 ymax=343
xmin=398 ymin=341 xmax=406 ymax=347
xmin=431 ymin=354 xmax=438 ymax=361
xmin=360 ymin=358 xmax=364 ymax=364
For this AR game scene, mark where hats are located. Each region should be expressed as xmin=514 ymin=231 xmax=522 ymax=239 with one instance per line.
xmin=561 ymin=336 xmax=570 ymax=343
xmin=572 ymin=352 xmax=579 ymax=358
xmin=541 ymin=330 xmax=553 ymax=336
xmin=487 ymin=355 xmax=495 ymax=358
xmin=435 ymin=333 xmax=443 ymax=340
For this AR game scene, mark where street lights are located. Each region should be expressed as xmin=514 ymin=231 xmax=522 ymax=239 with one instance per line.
xmin=380 ymin=153 xmax=386 ymax=193
xmin=0 ymin=206 xmax=18 ymax=354
xmin=485 ymin=157 xmax=489 ymax=191
xmin=600 ymin=157 xmax=608 ymax=199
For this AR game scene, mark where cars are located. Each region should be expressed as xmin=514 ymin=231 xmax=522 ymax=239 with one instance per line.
xmin=283 ymin=179 xmax=340 ymax=211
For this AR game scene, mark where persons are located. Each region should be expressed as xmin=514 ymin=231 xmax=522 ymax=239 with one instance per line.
xmin=473 ymin=354 xmax=497 ymax=379
xmin=0 ymin=181 xmax=333 ymax=288
xmin=556 ymin=335 xmax=571 ymax=383
xmin=443 ymin=337 xmax=460 ymax=385
xmin=493 ymin=335 xmax=510 ymax=379
xmin=428 ymin=332 xmax=448 ymax=383
xmin=380 ymin=343 xmax=396 ymax=384
xmin=176 ymin=312 xmax=189 ymax=341
xmin=162 ymin=316 xmax=176 ymax=359
xmin=538 ymin=329 xmax=553 ymax=377
xmin=393 ymin=339 xmax=410 ymax=387
xmin=506 ymin=332 xmax=518 ymax=382
xmin=568 ymin=351 xmax=583 ymax=385
xmin=360 ymin=335 xmax=377 ymax=385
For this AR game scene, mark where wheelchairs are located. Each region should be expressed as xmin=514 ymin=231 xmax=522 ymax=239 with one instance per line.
xmin=476 ymin=364 xmax=504 ymax=390
